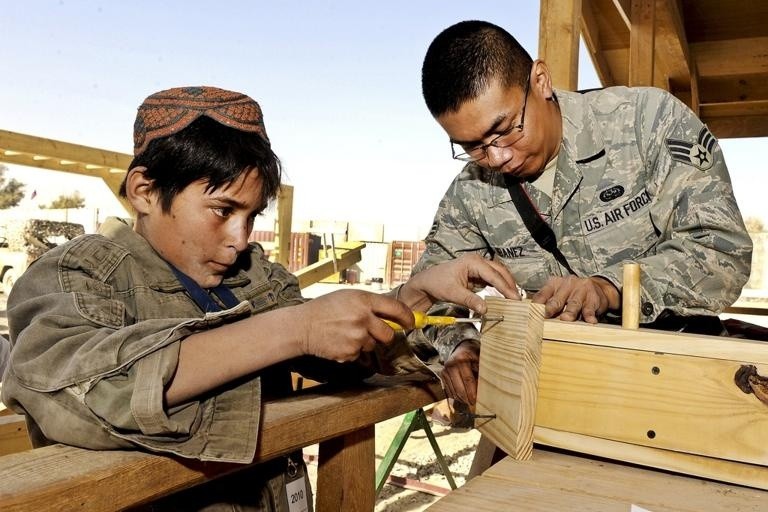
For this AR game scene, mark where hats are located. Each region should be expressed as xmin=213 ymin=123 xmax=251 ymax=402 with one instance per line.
xmin=132 ymin=86 xmax=271 ymax=155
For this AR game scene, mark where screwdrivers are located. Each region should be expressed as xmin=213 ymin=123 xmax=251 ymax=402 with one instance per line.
xmin=377 ymin=310 xmax=504 ymax=332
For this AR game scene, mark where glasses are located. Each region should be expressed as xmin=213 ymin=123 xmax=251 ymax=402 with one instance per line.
xmin=449 ymin=76 xmax=531 ymax=162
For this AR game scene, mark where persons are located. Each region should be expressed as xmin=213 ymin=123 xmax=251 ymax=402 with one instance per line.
xmin=0 ymin=85 xmax=522 ymax=512
xmin=412 ymin=19 xmax=753 ymax=411
